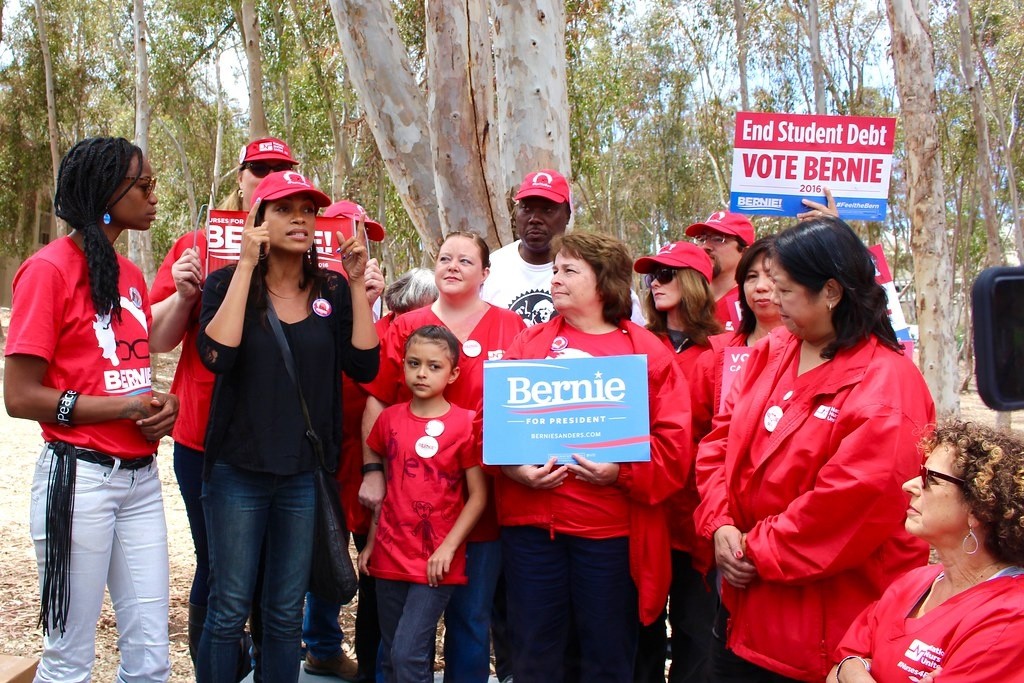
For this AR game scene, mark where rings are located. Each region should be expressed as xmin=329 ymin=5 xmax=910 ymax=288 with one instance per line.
xmin=818 ymin=212 xmax=822 ymax=217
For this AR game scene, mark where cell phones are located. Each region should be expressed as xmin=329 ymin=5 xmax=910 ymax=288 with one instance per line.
xmin=973 ymin=266 xmax=1024 ymax=411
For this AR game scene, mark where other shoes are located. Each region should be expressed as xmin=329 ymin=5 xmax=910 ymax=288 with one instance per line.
xmin=303 ymin=647 xmax=358 ymax=682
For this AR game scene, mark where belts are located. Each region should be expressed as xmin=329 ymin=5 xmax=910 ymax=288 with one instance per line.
xmin=54 ymin=444 xmax=158 ymax=471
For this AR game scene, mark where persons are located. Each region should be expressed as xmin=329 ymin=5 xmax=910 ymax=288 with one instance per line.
xmin=3 ymin=137 xmax=178 ymax=683
xmin=825 ymin=420 xmax=1024 ymax=683
xmin=149 ymin=134 xmax=840 ymax=683
xmin=696 ymin=214 xmax=939 ymax=683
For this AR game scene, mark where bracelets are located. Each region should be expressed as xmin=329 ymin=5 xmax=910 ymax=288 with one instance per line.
xmin=836 ymin=655 xmax=869 ymax=683
xmin=360 ymin=463 xmax=383 ymax=475
xmin=56 ymin=388 xmax=80 ymax=428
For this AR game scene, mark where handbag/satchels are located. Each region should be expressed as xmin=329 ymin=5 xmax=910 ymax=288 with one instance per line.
xmin=308 ymin=469 xmax=359 ymax=605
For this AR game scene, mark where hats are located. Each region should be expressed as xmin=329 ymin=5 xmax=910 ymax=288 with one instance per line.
xmin=633 ymin=241 xmax=714 ymax=284
xmin=686 ymin=210 xmax=755 ymax=247
xmin=323 ymin=200 xmax=385 ymax=242
xmin=239 ymin=137 xmax=300 ymax=166
xmin=513 ymin=169 xmax=571 ymax=203
xmin=251 ymin=170 xmax=332 ymax=211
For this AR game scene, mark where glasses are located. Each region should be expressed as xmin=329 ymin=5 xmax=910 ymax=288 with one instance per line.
xmin=126 ymin=175 xmax=158 ymax=199
xmin=693 ymin=233 xmax=737 ymax=246
xmin=239 ymin=161 xmax=293 ymax=178
xmin=644 ymin=268 xmax=680 ymax=287
xmin=919 ymin=465 xmax=968 ymax=490
